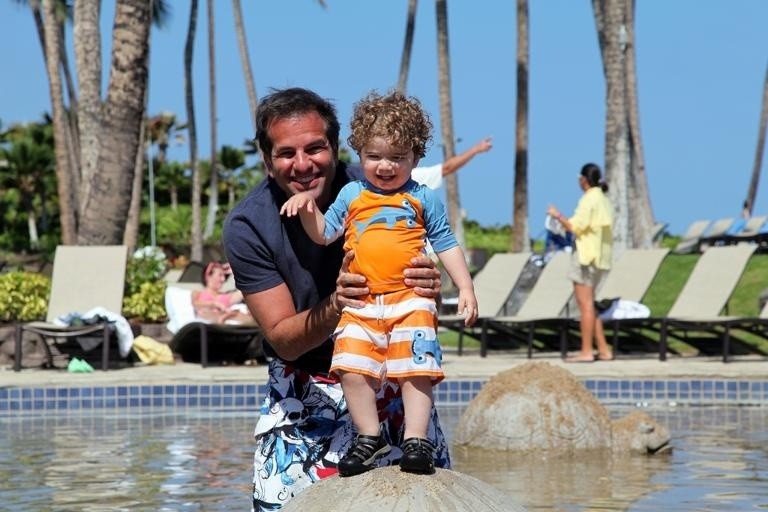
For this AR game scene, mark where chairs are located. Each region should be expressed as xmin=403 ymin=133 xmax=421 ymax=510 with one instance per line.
xmin=13 ymin=241 xmax=132 ymax=373
xmin=438 ymin=215 xmax=767 ymax=362
xmin=158 ymin=259 xmax=263 ymax=367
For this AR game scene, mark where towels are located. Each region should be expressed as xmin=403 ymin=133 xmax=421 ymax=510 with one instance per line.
xmin=602 ymin=297 xmax=652 ymax=322
xmin=164 ymin=286 xmax=247 ymax=332
xmin=81 ymin=307 xmax=133 ymax=358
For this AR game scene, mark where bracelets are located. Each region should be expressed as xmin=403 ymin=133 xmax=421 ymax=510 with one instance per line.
xmin=556 ymin=211 xmax=563 ymax=219
xmin=328 ymin=294 xmax=340 ymax=318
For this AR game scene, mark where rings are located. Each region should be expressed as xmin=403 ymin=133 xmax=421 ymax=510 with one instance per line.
xmin=430 ymin=278 xmax=434 ymax=287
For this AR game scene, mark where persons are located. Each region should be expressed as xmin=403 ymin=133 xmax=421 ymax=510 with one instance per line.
xmin=222 ymin=85 xmax=454 ymax=511
xmin=277 ymin=88 xmax=479 ymax=476
xmin=411 ymin=132 xmax=496 ymax=265
xmin=191 ymin=260 xmax=254 ymax=325
xmin=544 ymin=162 xmax=616 ymax=364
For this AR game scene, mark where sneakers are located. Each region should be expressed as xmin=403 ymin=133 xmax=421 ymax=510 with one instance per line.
xmin=400 ymin=437 xmax=437 ymax=474
xmin=338 ymin=422 xmax=392 ymax=475
